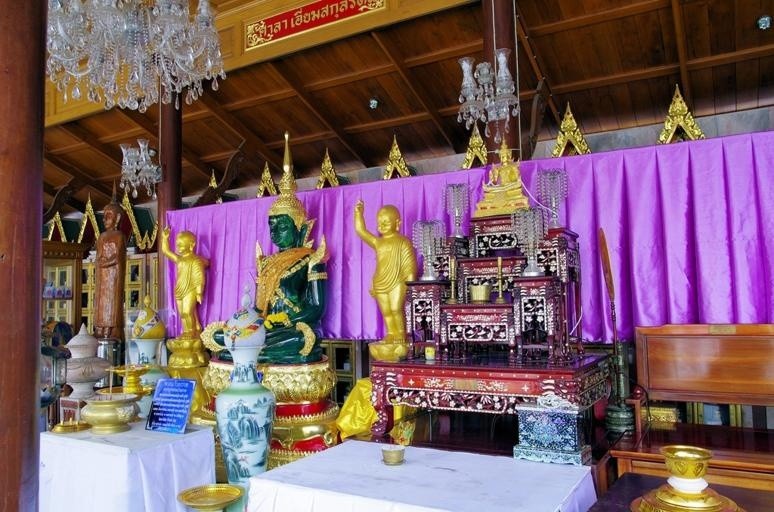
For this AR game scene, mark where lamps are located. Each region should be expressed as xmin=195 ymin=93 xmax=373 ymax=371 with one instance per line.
xmin=410 ymin=166 xmax=570 ymax=281
xmin=117 ymin=137 xmax=166 ymax=201
xmin=456 ymin=1 xmax=521 ymax=145
xmin=43 ymin=1 xmax=230 ymax=116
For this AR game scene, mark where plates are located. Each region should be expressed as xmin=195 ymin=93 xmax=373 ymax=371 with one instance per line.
xmin=176 ymin=483 xmax=244 ymax=511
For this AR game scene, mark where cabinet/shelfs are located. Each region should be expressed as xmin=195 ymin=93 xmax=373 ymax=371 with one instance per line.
xmin=41 ymin=239 xmax=90 ymax=336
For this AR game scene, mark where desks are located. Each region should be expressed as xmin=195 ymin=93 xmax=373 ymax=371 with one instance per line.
xmin=368 ymin=350 xmax=615 ymax=444
xmin=39 ymin=419 xmax=216 ymax=512
xmin=586 ymin=470 xmax=773 ymax=511
xmin=245 ymin=437 xmax=598 ymax=512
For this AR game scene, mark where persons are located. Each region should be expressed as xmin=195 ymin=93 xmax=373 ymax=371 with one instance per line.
xmin=90 ymin=202 xmax=129 ymax=339
xmin=353 ymin=198 xmax=418 ymax=343
xmin=157 ymin=222 xmax=206 ymax=339
xmin=212 ymin=195 xmax=331 ymax=364
xmin=482 ymin=133 xmax=521 ymax=193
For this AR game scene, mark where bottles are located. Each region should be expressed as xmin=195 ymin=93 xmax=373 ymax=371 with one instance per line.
xmin=213 ymin=346 xmax=275 ymax=484
xmin=629 ymin=445 xmax=746 ymax=511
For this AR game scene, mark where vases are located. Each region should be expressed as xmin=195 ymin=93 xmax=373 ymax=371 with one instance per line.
xmin=213 ymin=344 xmax=276 ymax=512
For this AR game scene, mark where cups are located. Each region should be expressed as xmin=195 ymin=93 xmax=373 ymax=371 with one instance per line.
xmin=382 ymin=443 xmax=405 ymax=464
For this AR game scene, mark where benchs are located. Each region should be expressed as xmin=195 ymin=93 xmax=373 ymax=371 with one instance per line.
xmin=607 ymin=321 xmax=774 ymax=490
